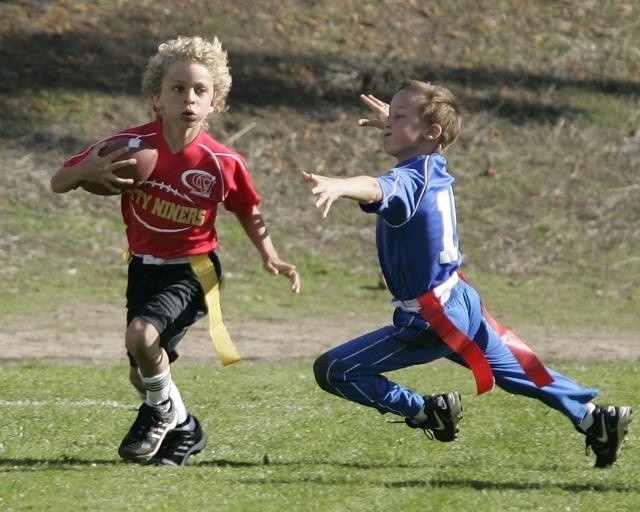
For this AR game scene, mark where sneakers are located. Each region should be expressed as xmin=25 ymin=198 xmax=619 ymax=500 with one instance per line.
xmin=406 ymin=392 xmax=462 ymax=442
xmin=586 ymin=404 xmax=633 ymax=468
xmin=148 ymin=415 xmax=208 ymax=468
xmin=118 ymin=398 xmax=178 ymax=462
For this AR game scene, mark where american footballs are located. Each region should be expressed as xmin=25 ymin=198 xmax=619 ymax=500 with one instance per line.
xmin=80 ymin=138 xmax=158 ymax=196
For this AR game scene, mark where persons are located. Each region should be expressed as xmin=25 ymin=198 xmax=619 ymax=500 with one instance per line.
xmin=52 ymin=36 xmax=300 ymax=468
xmin=302 ymin=80 xmax=632 ymax=469
